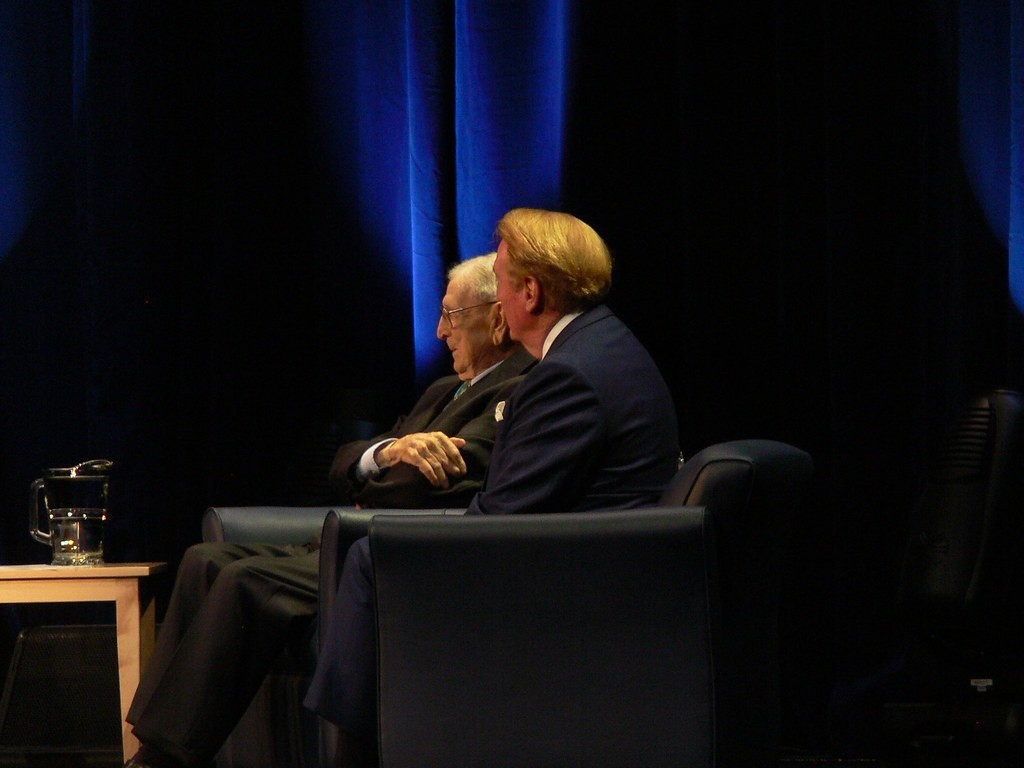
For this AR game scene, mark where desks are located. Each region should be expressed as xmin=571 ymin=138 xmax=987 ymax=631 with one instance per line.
xmin=0 ymin=562 xmax=169 ymax=764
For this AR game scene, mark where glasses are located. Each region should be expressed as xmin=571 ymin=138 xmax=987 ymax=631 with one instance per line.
xmin=441 ymin=301 xmax=497 ymax=327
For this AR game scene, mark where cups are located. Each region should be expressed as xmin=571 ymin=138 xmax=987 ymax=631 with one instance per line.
xmin=29 ymin=460 xmax=114 ymax=565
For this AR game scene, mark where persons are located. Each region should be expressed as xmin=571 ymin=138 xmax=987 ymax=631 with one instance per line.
xmin=118 ymin=251 xmax=542 ymax=768
xmin=302 ymin=208 xmax=675 ymax=740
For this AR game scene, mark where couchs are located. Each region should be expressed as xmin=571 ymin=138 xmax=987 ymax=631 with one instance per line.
xmin=200 ymin=439 xmax=812 ymax=768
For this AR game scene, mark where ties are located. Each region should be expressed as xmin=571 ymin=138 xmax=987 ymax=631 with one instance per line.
xmin=453 ymin=380 xmax=471 ymax=401
